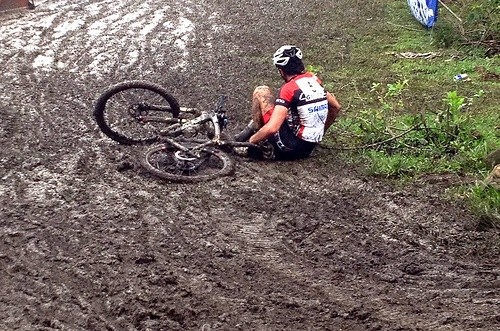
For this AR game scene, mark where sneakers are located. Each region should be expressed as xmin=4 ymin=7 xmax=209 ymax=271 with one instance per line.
xmin=234 ymin=126 xmax=259 ymax=141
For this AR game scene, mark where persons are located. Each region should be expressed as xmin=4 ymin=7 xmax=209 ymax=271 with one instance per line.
xmin=207 ymin=45 xmax=342 ymax=161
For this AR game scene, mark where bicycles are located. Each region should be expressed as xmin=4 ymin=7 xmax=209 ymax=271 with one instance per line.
xmin=92 ymin=79 xmax=277 ymax=184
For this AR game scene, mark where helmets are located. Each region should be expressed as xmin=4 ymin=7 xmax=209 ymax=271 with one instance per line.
xmin=273 ymin=45 xmax=304 ymax=67
